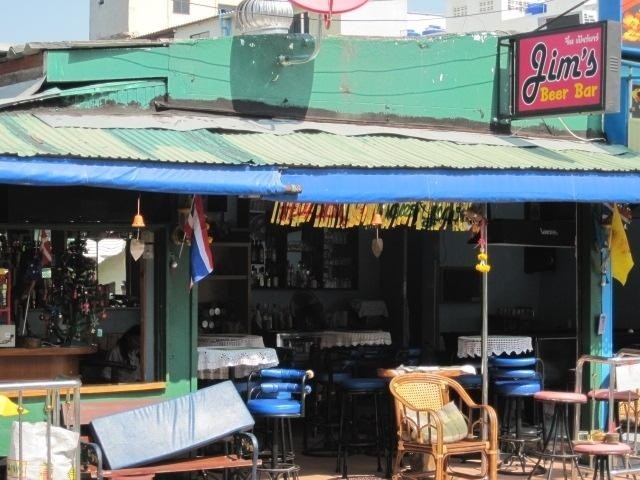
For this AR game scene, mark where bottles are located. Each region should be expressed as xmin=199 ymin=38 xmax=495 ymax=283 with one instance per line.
xmin=283 ymin=259 xmax=318 ymax=289
xmin=251 ymin=240 xmax=259 ymax=265
xmin=258 ymin=239 xmax=276 ymax=264
xmin=265 ymin=305 xmax=272 ymax=330
xmin=272 ymin=307 xmax=292 ymax=330
xmin=197 ymin=301 xmax=245 ymax=332
xmin=322 ymin=228 xmax=353 ymax=288
xmin=498 ymin=308 xmax=533 ymax=330
xmin=252 ymin=266 xmax=279 ymax=289
xmin=255 ymin=304 xmax=262 ymax=331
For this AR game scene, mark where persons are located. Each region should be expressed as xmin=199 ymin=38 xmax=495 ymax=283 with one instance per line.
xmin=101 ymin=325 xmax=142 ymax=384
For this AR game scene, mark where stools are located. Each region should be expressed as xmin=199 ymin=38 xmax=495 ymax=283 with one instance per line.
xmin=574 ymin=440 xmax=630 ymax=480
xmin=587 ymin=389 xmax=639 ymax=469
xmin=528 ymin=391 xmax=587 ymax=480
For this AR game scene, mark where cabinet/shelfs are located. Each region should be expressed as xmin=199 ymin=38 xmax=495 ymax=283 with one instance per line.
xmin=199 ymin=243 xmax=251 ymax=335
xmin=238 ymin=199 xmax=410 ymax=350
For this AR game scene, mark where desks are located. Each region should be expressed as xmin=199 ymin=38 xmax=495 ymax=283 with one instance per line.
xmin=0 ymin=341 xmax=97 ymax=381
xmin=129 ymin=345 xmax=280 ymax=383
xmin=276 ymin=331 xmax=392 ymax=457
xmin=457 ymin=335 xmax=534 ymax=364
xmin=198 ymin=335 xmax=264 ymax=348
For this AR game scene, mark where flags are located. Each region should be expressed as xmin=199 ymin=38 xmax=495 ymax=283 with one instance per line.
xmin=609 ymin=202 xmax=635 ymax=286
xmin=184 ymin=194 xmax=214 ymax=293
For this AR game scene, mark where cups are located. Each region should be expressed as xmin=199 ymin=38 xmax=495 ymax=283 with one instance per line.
xmin=606 ymin=433 xmax=619 ymax=444
xmin=577 ymin=431 xmax=588 ymax=442
xmin=591 ymin=427 xmax=603 ymax=442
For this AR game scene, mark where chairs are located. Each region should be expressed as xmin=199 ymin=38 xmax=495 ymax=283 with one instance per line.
xmin=79 ymin=359 xmax=136 ymax=386
xmin=246 ymin=369 xmax=314 ymax=480
xmin=450 ymin=357 xmax=493 ymax=435
xmin=390 ymin=373 xmax=499 ymax=480
xmin=302 ymin=364 xmax=353 ymax=456
xmin=336 ymin=358 xmax=392 ymax=479
xmin=490 ymin=359 xmax=546 ymax=474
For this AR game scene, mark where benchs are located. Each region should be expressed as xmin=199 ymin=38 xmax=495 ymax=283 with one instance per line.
xmin=60 ymin=398 xmax=262 ymax=480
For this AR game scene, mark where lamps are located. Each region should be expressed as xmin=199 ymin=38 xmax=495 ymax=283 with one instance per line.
xmin=172 ymin=209 xmax=190 ymax=246
